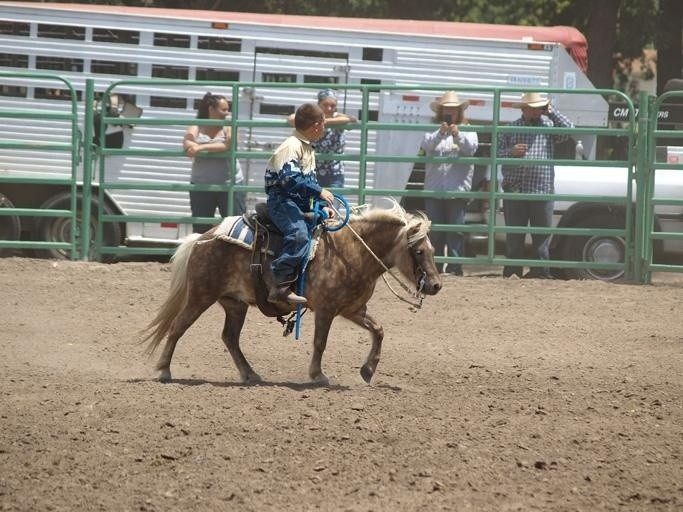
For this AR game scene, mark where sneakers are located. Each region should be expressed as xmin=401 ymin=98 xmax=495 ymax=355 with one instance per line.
xmin=267 ymin=285 xmax=308 ymax=304
xmin=504 ymin=271 xmax=557 ymax=281
xmin=445 ymin=268 xmax=464 ymax=277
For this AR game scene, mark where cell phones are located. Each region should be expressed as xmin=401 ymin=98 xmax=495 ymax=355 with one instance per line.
xmin=445 ymin=113 xmax=452 ymax=127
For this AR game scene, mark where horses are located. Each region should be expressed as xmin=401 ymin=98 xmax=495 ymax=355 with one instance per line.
xmin=135 ymin=193 xmax=443 ymax=388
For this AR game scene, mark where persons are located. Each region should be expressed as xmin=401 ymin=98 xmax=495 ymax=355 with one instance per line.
xmin=182 ymin=90 xmax=246 ymax=236
xmin=495 ymin=91 xmax=574 ymax=280
xmin=418 ymin=92 xmax=480 ymax=277
xmin=286 ymin=90 xmax=359 ymax=212
xmin=262 ymin=103 xmax=334 ymax=305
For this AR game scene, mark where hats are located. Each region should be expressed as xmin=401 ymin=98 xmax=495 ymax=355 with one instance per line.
xmin=429 ymin=90 xmax=469 ymax=113
xmin=511 ymin=92 xmax=551 ymax=108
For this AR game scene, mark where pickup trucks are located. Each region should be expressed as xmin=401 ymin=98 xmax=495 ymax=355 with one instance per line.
xmin=483 ymin=145 xmax=683 ymax=282
